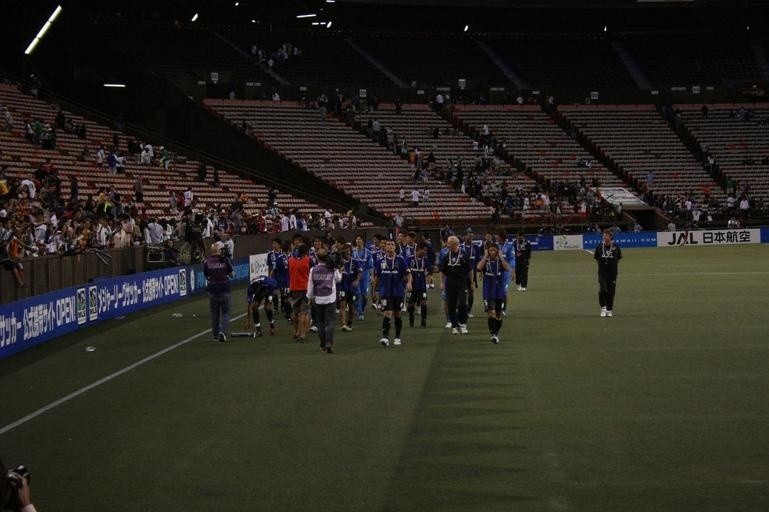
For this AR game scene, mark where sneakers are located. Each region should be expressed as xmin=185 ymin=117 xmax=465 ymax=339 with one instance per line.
xmin=320 ymin=344 xmax=332 ymax=353
xmin=517 ymin=284 xmax=526 ymax=291
xmin=213 ymin=332 xmax=226 ymax=342
xmin=380 ymin=338 xmax=389 ymax=346
xmin=445 ymin=311 xmax=506 ymax=344
xmin=371 ymin=284 xmax=435 ymax=315
xmin=394 ymin=338 xmax=401 ymax=345
xmin=600 ymin=306 xmax=613 ymax=317
xmin=253 ymin=306 xmax=364 ymax=342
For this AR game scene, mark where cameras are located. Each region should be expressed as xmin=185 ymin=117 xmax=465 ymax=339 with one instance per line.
xmin=8 ymin=465 xmax=32 ymax=491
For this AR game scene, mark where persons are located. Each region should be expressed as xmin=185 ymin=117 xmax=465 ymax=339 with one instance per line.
xmin=0 ymin=463 xmax=37 ymax=512
xmin=1 ymin=43 xmax=768 ymax=354
